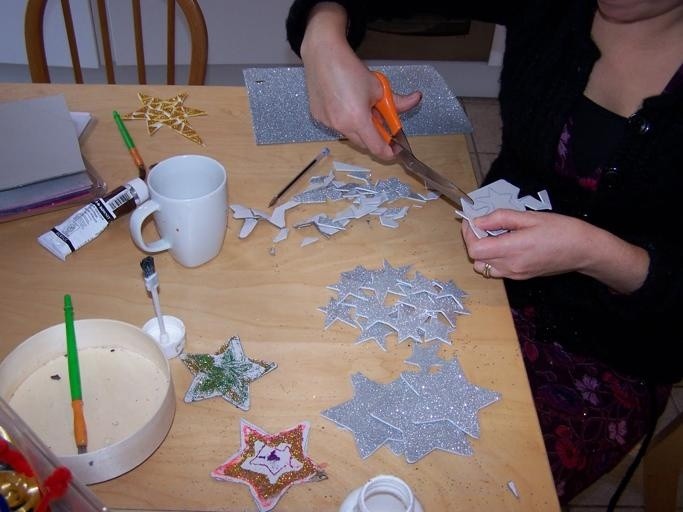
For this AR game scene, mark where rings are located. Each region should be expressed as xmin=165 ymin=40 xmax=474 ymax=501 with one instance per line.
xmin=482 ymin=263 xmax=492 ymax=278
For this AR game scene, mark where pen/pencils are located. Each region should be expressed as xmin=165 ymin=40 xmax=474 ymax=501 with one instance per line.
xmin=268 ymin=149 xmax=329 ymax=208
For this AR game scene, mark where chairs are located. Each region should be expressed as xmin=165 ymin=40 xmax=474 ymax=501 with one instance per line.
xmin=23 ymin=1 xmax=209 ymax=85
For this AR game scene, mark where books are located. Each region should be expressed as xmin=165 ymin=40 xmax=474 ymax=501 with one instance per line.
xmin=0 ymin=91 xmax=107 ymax=225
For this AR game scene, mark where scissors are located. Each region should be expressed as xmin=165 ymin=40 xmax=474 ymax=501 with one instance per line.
xmin=371 ymin=72 xmax=474 ymax=206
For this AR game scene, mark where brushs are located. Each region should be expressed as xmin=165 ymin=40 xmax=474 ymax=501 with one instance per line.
xmin=139 ymin=256 xmax=185 ymax=360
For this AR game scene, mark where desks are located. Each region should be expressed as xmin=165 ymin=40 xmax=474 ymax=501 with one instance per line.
xmin=1 ymin=83 xmax=558 ymax=512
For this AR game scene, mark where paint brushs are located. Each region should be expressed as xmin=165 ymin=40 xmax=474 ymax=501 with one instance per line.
xmin=113 ymin=111 xmax=146 ymax=180
xmin=63 ymin=295 xmax=87 ymax=454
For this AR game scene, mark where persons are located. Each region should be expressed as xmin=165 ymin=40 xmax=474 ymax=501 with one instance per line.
xmin=282 ymin=0 xmax=681 ymax=510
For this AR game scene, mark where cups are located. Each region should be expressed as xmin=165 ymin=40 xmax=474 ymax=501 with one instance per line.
xmin=127 ymin=151 xmax=229 ymax=270
xmin=140 ymin=316 xmax=187 ymax=357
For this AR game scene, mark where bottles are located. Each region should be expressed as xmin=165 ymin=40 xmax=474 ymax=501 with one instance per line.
xmin=335 ymin=473 xmax=425 ymax=512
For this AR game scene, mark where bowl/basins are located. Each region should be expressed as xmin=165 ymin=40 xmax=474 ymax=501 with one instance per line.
xmin=0 ymin=319 xmax=176 ymax=488
